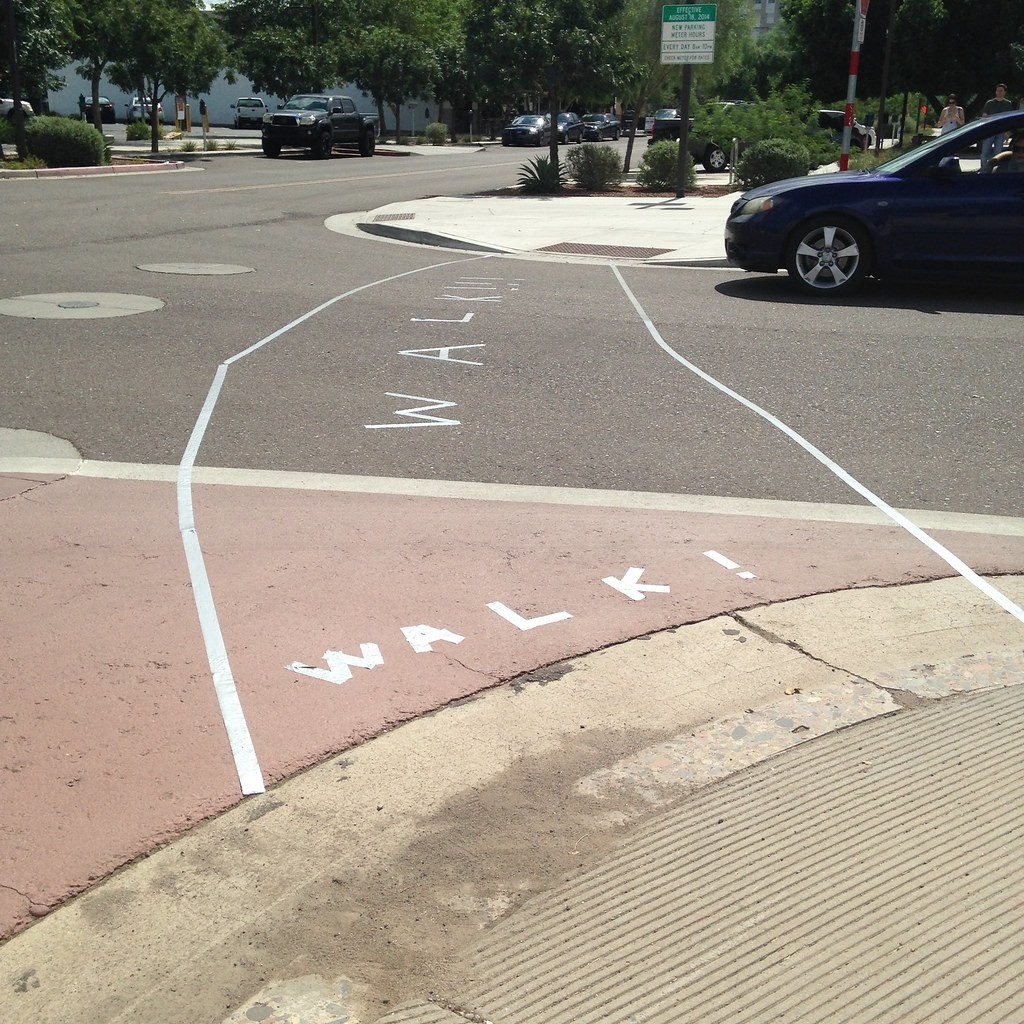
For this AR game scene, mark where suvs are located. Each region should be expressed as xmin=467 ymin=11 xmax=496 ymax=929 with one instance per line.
xmin=229 ymin=97 xmax=268 ymax=129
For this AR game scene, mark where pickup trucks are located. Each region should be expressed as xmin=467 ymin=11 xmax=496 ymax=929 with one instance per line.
xmin=647 ymin=117 xmax=752 ymax=174
xmin=262 ymin=94 xmax=381 ymax=161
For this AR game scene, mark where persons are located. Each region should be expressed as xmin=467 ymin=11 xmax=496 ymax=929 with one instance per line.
xmin=981 ymin=84 xmax=1011 ymax=168
xmin=938 ymin=94 xmax=965 ymax=157
xmin=938 ymin=130 xmax=1024 ymax=175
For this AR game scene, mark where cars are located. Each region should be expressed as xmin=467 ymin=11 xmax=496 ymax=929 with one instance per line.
xmin=0 ymin=98 xmax=35 ymax=126
xmin=77 ymin=97 xmax=115 ymax=125
xmin=817 ymin=110 xmax=877 ymax=152
xmin=125 ymin=96 xmax=165 ymax=125
xmin=502 ymin=114 xmax=551 ymax=148
xmin=653 ymin=108 xmax=682 ymax=119
xmin=724 ymin=109 xmax=1024 ymax=297
xmin=582 ymin=112 xmax=623 ymax=142
xmin=556 ymin=112 xmax=586 ymax=144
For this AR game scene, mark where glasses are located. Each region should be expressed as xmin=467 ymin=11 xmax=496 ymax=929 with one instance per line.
xmin=948 ymin=102 xmax=956 ymax=105
